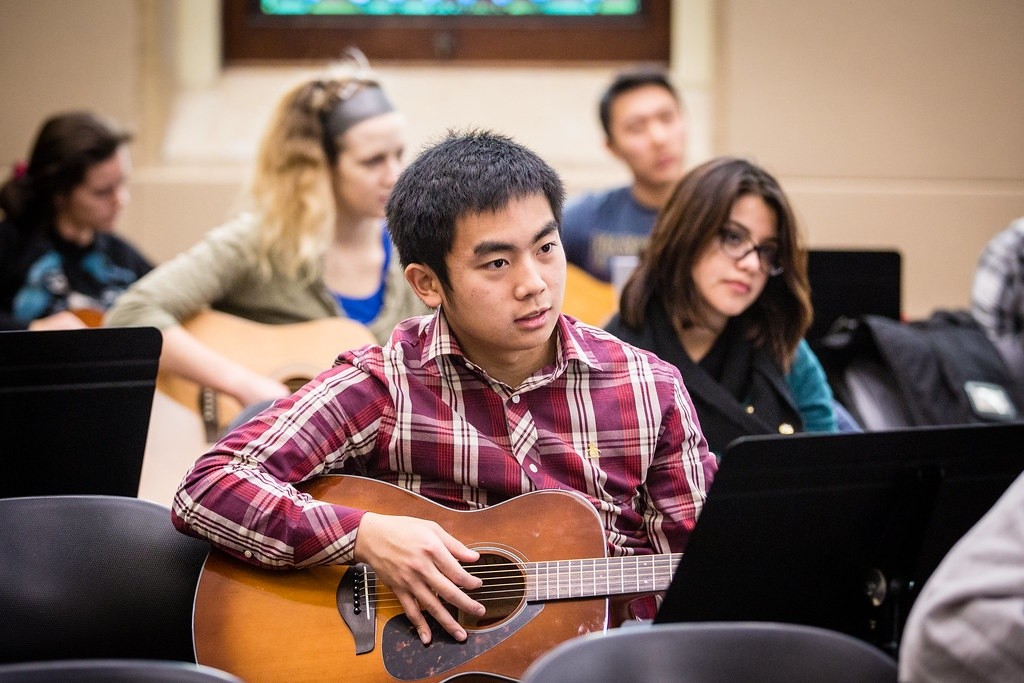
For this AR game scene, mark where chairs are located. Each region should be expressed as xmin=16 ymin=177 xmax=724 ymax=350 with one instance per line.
xmin=0 ymin=334 xmax=1024 ymax=683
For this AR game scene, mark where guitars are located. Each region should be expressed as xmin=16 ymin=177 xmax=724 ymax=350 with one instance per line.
xmin=157 ymin=309 xmax=379 ymax=444
xmin=190 ymin=474 xmax=684 ymax=683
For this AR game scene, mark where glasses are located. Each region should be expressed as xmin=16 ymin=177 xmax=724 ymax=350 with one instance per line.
xmin=716 ymin=227 xmax=790 ymax=277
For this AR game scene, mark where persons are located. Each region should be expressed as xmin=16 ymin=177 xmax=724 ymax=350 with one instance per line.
xmin=972 ymin=218 xmax=1024 ymax=376
xmin=0 ymin=111 xmax=154 ymax=331
xmin=107 ymin=69 xmax=435 ymax=408
xmin=562 ymin=72 xmax=687 ymax=285
xmin=171 ymin=130 xmax=717 ymax=628
xmin=602 ymin=157 xmax=838 ymax=459
